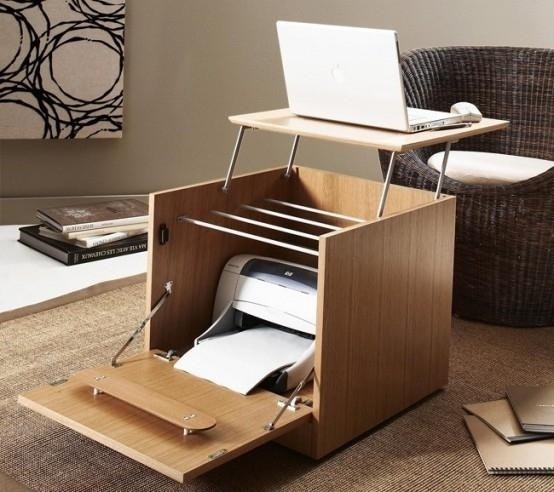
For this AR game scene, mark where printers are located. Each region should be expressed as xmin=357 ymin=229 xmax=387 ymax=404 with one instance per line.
xmin=173 ymin=255 xmax=319 ymax=394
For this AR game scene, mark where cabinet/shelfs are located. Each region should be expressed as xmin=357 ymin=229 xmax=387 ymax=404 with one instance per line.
xmin=16 ymin=106 xmax=510 ymax=485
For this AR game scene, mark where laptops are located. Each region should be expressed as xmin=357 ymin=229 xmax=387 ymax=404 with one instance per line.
xmin=276 ymin=21 xmax=471 ymax=134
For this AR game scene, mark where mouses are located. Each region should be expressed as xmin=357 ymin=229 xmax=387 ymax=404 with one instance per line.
xmin=451 ymin=102 xmax=481 ymax=122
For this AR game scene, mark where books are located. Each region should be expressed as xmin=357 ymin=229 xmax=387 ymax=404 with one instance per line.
xmin=505 ymin=383 xmax=554 ymax=433
xmin=461 ymin=414 xmax=554 ymax=475
xmin=16 ymin=198 xmax=149 ymax=266
xmin=461 ymin=397 xmax=554 ymax=444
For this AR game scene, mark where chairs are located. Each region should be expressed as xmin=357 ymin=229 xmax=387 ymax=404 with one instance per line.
xmin=378 ymin=46 xmax=554 ymax=328
